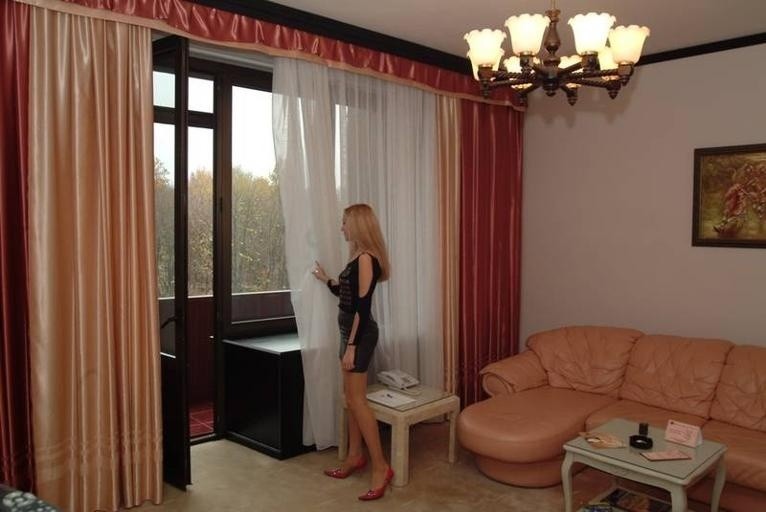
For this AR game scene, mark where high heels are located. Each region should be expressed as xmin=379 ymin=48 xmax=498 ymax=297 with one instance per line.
xmin=324 ymin=452 xmax=366 ymax=479
xmin=358 ymin=464 xmax=394 ymax=501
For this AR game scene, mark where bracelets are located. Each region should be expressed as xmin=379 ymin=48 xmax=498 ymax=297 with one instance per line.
xmin=348 ymin=343 xmax=356 ymax=346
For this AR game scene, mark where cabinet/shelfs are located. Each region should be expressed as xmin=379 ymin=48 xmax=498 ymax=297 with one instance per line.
xmin=221 ymin=314 xmax=400 ymax=462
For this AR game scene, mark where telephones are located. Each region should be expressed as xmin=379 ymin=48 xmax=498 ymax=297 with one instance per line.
xmin=376 ymin=369 xmax=419 ymax=389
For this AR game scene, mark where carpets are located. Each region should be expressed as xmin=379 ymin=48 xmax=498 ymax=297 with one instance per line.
xmin=576 ymin=483 xmax=694 ymax=512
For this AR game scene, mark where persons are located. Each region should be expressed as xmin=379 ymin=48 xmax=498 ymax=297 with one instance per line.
xmin=309 ymin=203 xmax=394 ymax=501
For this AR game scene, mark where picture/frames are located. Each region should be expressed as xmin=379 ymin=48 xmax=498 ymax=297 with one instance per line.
xmin=693 ymin=143 xmax=766 ymax=248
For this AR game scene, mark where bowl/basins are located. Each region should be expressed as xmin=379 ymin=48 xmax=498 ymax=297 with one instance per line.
xmin=630 ymin=435 xmax=654 ymax=449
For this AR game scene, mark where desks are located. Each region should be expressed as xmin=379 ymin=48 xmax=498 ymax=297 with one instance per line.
xmin=560 ymin=418 xmax=729 ymax=512
xmin=337 ymin=381 xmax=462 ymax=487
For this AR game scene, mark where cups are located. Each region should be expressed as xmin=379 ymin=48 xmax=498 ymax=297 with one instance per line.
xmin=639 ymin=423 xmax=649 ymax=435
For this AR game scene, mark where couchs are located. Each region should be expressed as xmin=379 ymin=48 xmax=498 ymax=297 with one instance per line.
xmin=457 ymin=325 xmax=766 ymax=512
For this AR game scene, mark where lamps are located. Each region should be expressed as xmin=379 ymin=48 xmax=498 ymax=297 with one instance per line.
xmin=462 ymin=0 xmax=650 ymax=105
